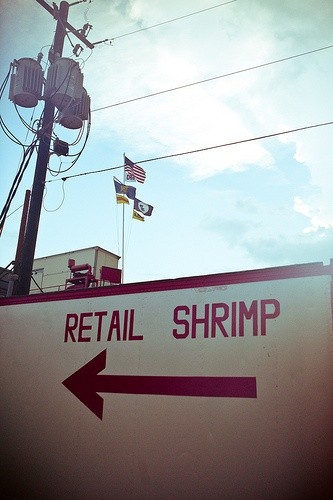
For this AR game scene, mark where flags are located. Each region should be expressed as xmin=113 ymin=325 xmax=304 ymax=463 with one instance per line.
xmin=134 ymin=198 xmax=153 ymax=216
xmin=115 ymin=179 xmax=136 ymax=200
xmin=132 ymin=210 xmax=144 ymax=222
xmin=116 ymin=194 xmax=129 ymax=205
xmin=124 ymin=156 xmax=146 ymax=184
